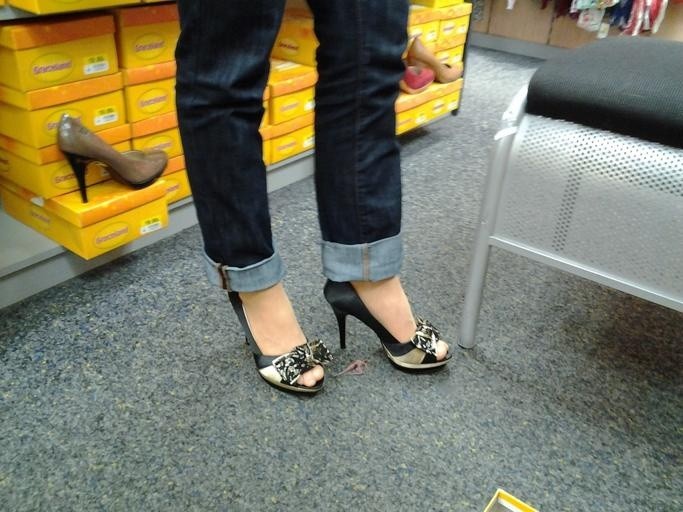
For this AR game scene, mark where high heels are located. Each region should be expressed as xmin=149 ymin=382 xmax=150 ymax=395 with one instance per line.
xmin=324 ymin=279 xmax=452 ymax=374
xmin=57 ymin=114 xmax=168 ymax=203
xmin=227 ymin=291 xmax=336 ymax=398
xmin=399 ymin=38 xmax=459 ymax=94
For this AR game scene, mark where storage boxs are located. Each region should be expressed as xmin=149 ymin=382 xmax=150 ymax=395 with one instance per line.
xmin=118 ymin=5 xmax=186 ymax=64
xmin=129 ymin=116 xmax=182 ymax=163
xmin=1 ymin=12 xmax=120 ymax=94
xmin=123 ymin=63 xmax=176 ymax=121
xmin=2 ymin=73 xmax=126 ymax=147
xmin=257 ymin=3 xmax=471 ymax=167
xmin=164 ymin=156 xmax=191 ymax=201
xmin=1 ymin=124 xmax=131 ymax=200
xmin=1 ymin=176 xmax=168 ymax=259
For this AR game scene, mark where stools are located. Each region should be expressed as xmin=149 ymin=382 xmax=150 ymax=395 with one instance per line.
xmin=455 ymin=36 xmax=682 ymax=353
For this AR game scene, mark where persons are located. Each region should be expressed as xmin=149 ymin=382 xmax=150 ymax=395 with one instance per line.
xmin=173 ymin=0 xmax=453 ymax=397
